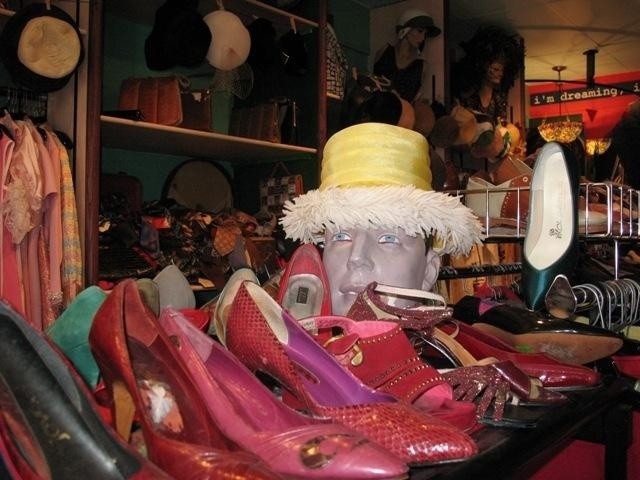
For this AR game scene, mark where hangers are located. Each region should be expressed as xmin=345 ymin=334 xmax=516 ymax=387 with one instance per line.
xmin=570 ymin=276 xmax=639 ymax=358
xmin=432 ymin=259 xmax=525 ymax=308
xmin=1 ymin=85 xmax=74 ymax=153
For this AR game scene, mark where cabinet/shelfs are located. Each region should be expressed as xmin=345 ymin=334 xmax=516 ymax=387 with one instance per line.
xmin=433 ymin=186 xmax=636 ymax=278
xmin=1 ymin=0 xmax=329 ymax=342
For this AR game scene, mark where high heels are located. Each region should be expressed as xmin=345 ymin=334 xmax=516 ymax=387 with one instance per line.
xmin=0 ymin=241 xmax=626 ymax=480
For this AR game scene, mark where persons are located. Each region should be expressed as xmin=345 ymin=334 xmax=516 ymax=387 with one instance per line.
xmin=280 ymin=123 xmax=488 ymax=318
xmin=370 ymin=5 xmax=442 ymax=103
xmin=461 ymin=48 xmax=522 ymax=125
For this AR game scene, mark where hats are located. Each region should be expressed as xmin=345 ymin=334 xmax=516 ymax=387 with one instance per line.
xmin=145 ymin=1 xmax=312 ymax=79
xmin=278 ymin=123 xmax=486 ymax=257
xmin=0 ymin=2 xmax=86 ymax=92
xmin=370 ymin=89 xmax=510 ymax=189
xmin=396 ymin=9 xmax=441 ymax=38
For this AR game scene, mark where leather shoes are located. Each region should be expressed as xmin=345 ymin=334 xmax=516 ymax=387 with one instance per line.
xmin=520 ymin=140 xmax=581 ymax=309
xmin=465 ymin=154 xmax=638 ymax=227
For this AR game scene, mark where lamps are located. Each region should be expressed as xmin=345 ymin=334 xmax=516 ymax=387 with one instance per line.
xmin=582 ymin=138 xmax=612 ymax=157
xmin=537 ymin=65 xmax=583 ymax=143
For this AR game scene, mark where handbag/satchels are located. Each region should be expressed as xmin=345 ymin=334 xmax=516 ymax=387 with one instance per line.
xmin=118 ymin=73 xmax=303 ymax=146
xmin=258 ymin=160 xmax=304 ymax=215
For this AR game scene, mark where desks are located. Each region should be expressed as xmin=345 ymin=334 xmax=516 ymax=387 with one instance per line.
xmin=403 ymin=360 xmax=639 ymax=479
